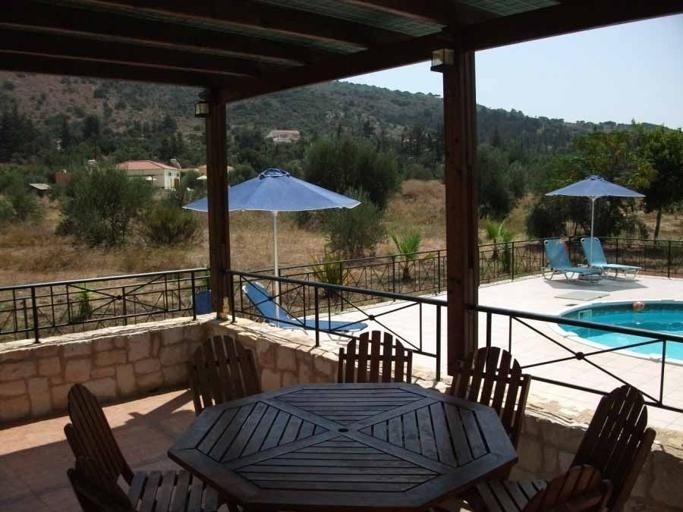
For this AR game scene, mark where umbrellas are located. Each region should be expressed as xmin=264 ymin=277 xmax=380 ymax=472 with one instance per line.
xmin=544 ymin=178 xmax=648 ymax=269
xmin=178 ymin=170 xmax=363 ymax=326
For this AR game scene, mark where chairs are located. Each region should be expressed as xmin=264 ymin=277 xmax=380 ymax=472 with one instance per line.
xmin=544 ymin=237 xmax=642 ymax=284
xmin=195 ymin=282 xmax=368 ymax=344
